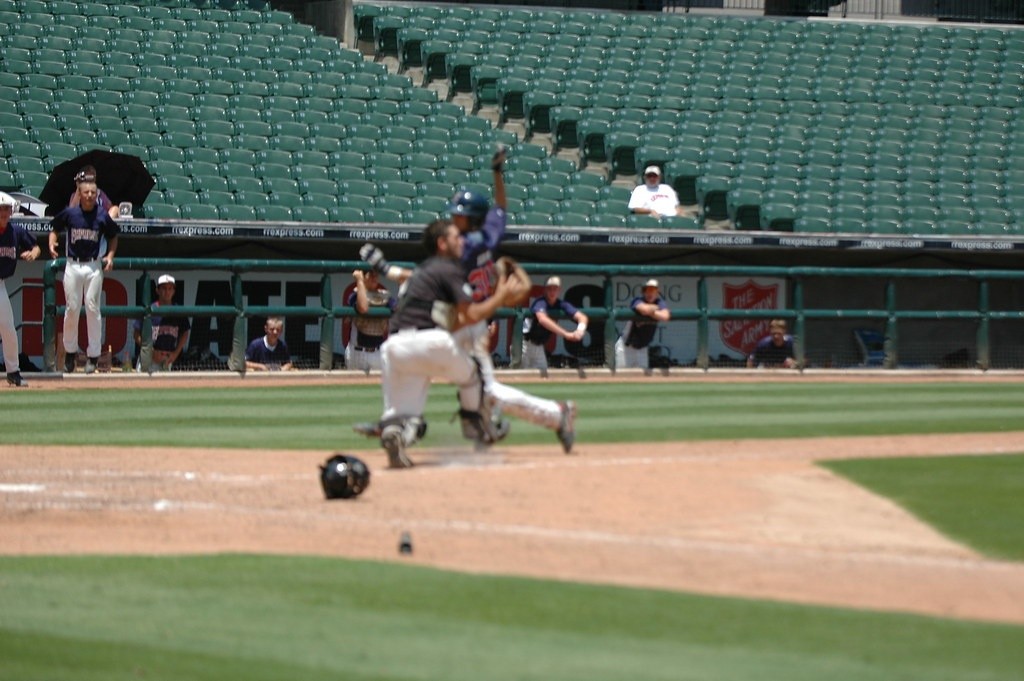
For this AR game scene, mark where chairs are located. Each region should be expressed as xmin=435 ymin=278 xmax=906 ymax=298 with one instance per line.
xmin=0 ymin=1 xmax=1023 ymax=237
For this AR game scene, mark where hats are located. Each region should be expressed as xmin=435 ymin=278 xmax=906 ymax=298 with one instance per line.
xmin=642 ymin=279 xmax=658 ymax=288
xmin=0 ymin=191 xmax=16 ymax=206
xmin=544 ymin=275 xmax=561 ymax=287
xmin=157 ymin=274 xmax=176 ymax=287
xmin=645 ymin=165 xmax=661 ymax=176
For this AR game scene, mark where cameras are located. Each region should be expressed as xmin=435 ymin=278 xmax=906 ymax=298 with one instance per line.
xmin=78 ymin=172 xmax=95 ymax=181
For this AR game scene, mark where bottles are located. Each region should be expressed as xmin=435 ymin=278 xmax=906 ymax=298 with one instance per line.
xmin=160 ymin=352 xmax=169 ymax=371
xmin=122 ymin=351 xmax=132 ymax=372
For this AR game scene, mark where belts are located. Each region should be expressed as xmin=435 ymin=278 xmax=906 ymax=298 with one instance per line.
xmin=354 ymin=347 xmax=379 ymax=352
xmin=391 ymin=325 xmax=435 ymax=334
xmin=73 ymin=256 xmax=97 ymax=261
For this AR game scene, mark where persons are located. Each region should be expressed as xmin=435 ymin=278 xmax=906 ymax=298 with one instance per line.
xmin=352 ymin=147 xmax=578 ymax=455
xmin=48 ymin=181 xmax=121 ymax=373
xmin=133 ymin=273 xmax=191 ymax=373
xmin=68 ymin=164 xmax=119 ymax=220
xmin=627 ymin=165 xmax=687 ymax=220
xmin=0 ymin=191 xmax=41 ymax=387
xmin=245 ymin=314 xmax=293 ymax=372
xmin=344 ymin=264 xmax=401 ymax=372
xmin=377 ymin=219 xmax=526 ymax=468
xmin=745 ymin=316 xmax=808 ymax=370
xmin=614 ymin=278 xmax=672 ymax=370
xmin=518 ymin=274 xmax=589 ymax=369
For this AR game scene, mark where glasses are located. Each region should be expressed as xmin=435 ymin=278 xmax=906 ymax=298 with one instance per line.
xmin=647 ymin=174 xmax=657 ymax=178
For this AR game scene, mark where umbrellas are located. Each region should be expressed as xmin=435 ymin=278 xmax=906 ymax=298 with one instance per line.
xmin=38 ymin=149 xmax=157 ymax=216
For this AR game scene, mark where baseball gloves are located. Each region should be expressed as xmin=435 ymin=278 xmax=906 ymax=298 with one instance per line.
xmin=496 ymin=256 xmax=533 ymax=308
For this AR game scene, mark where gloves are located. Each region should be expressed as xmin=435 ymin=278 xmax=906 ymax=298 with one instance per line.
xmin=492 ymin=143 xmax=507 ymax=171
xmin=360 ymin=243 xmax=390 ymax=276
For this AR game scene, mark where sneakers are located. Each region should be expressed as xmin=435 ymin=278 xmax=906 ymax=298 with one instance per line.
xmin=354 ymin=422 xmax=382 ymax=436
xmin=463 ymin=419 xmax=493 ymax=445
xmin=85 ymin=357 xmax=98 ymax=373
xmin=64 ymin=352 xmax=77 ymax=373
xmin=555 ymin=400 xmax=576 ymax=453
xmin=7 ymin=371 xmax=27 ymax=387
xmin=380 ymin=424 xmax=414 ymax=467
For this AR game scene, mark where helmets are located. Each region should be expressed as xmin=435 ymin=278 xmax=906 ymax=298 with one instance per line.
xmin=321 ymin=456 xmax=368 ymax=498
xmin=448 ymin=190 xmax=489 ymax=223
